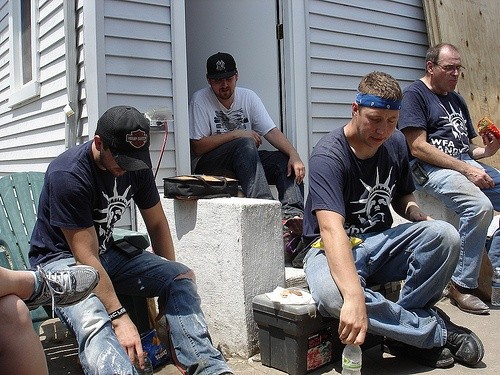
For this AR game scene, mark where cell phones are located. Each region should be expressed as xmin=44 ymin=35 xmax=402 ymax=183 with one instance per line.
xmin=112 ymin=239 xmax=143 ymax=259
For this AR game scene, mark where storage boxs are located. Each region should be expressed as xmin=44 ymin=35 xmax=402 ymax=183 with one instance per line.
xmin=252 ymin=286 xmax=384 ymax=375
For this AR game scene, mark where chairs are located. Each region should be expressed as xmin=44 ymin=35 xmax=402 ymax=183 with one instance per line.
xmin=0 ymin=171 xmax=160 ymax=336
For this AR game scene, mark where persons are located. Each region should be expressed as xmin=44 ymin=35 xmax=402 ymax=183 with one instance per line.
xmin=302 ymin=72 xmax=485 ymax=367
xmin=0 ymin=265 xmax=100 ymax=375
xmin=189 ymin=52 xmax=306 ymax=209
xmin=396 ymin=42 xmax=500 ymax=314
xmin=27 ymin=105 xmax=234 ymax=375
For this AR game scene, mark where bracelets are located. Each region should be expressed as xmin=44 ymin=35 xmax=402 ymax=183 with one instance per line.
xmin=108 ymin=306 xmax=126 ymax=320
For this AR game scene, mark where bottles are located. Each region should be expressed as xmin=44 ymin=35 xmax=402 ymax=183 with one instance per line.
xmin=342 ymin=342 xmax=362 ymax=375
xmin=134 ymin=356 xmax=153 ymax=375
xmin=491 ymin=268 xmax=500 ymax=306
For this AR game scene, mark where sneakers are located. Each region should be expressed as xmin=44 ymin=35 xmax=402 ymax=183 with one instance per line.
xmin=282 ymin=206 xmax=304 ymax=256
xmin=22 ymin=265 xmax=100 ymax=319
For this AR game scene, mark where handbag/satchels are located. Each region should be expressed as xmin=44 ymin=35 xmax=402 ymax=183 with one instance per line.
xmin=162 ymin=175 xmax=239 ymax=200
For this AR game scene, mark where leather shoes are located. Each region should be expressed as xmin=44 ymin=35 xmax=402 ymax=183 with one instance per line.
xmin=428 ymin=305 xmax=484 ymax=368
xmin=449 ymin=280 xmax=490 ymax=313
xmin=382 ymin=335 xmax=454 ymax=367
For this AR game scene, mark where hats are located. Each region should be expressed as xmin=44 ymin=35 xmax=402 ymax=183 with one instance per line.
xmin=97 ymin=105 xmax=152 ymax=171
xmin=206 ymin=52 xmax=237 ymax=79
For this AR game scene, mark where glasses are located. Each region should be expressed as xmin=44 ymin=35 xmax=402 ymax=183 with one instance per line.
xmin=430 ymin=60 xmax=465 ymax=72
